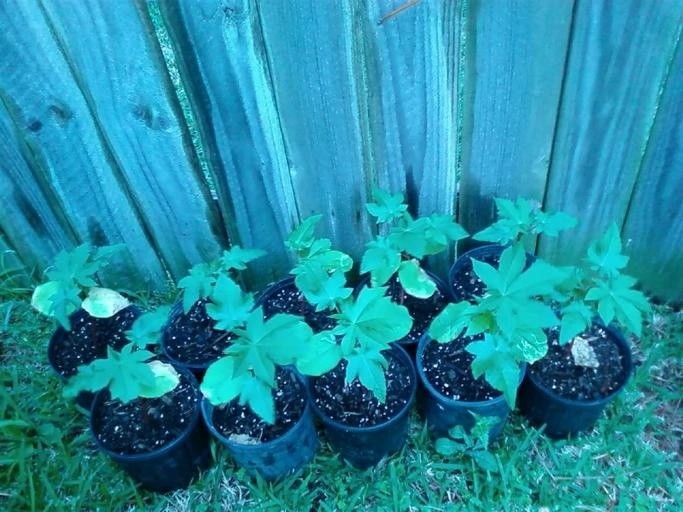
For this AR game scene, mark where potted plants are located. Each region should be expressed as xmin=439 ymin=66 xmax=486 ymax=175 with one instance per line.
xmin=355 ymin=185 xmax=472 ymax=349
xmin=295 ymin=254 xmax=418 ymax=472
xmin=199 ymin=273 xmax=346 ymax=482
xmin=30 ymin=239 xmax=156 ymax=414
xmin=416 ymin=234 xmax=566 ymax=445
xmin=159 ymin=238 xmax=268 ymax=377
xmin=520 ymin=221 xmax=653 ymax=439
xmin=61 ymin=303 xmax=207 ymax=492
xmin=449 ymin=191 xmax=579 ymax=313
xmin=255 ymin=213 xmax=355 ymax=345
